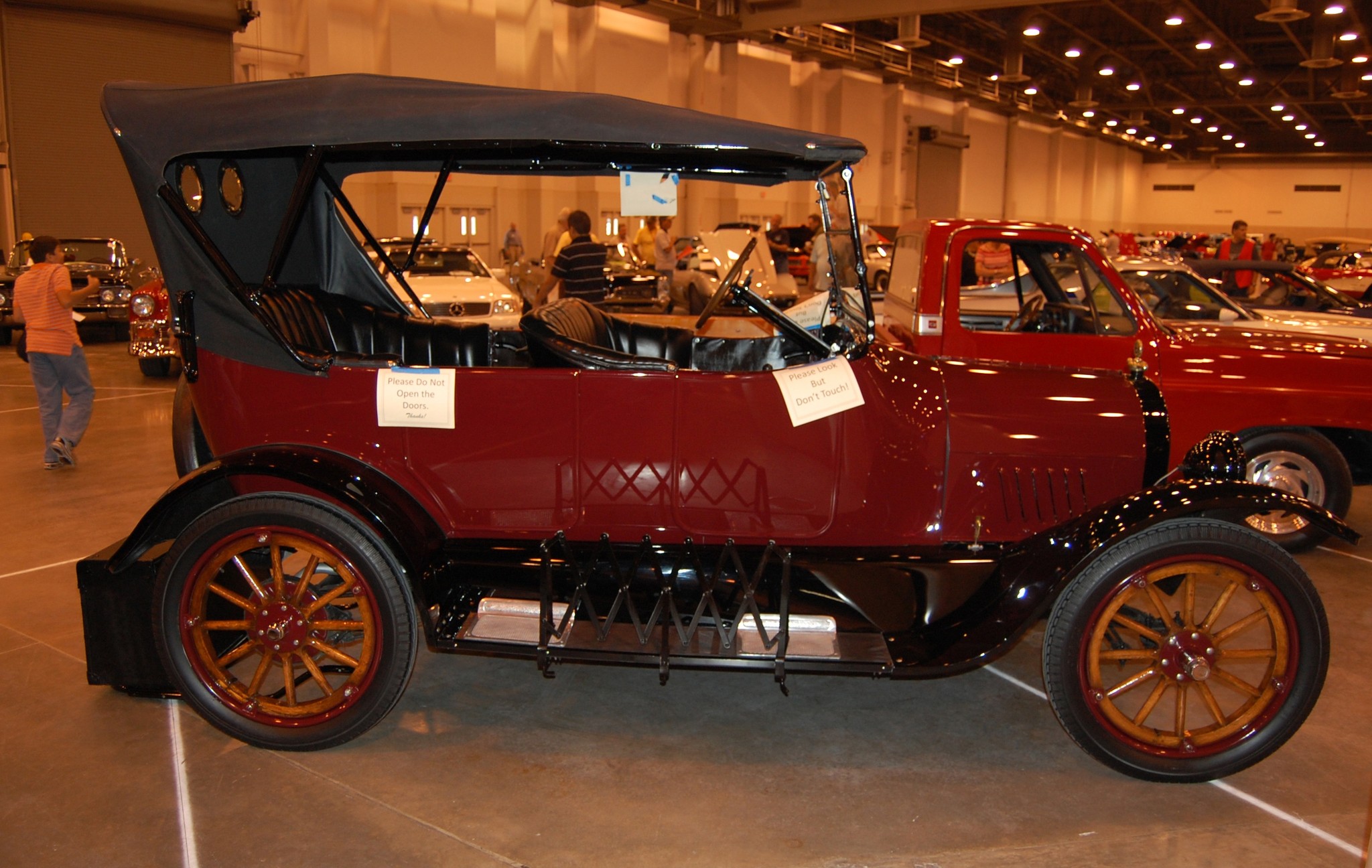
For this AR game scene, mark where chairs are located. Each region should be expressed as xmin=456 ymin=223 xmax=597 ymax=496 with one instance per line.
xmin=518 ymin=295 xmax=696 ymax=375
xmin=245 ymin=281 xmax=490 ymax=371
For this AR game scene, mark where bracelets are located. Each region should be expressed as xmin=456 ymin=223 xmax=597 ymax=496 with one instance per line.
xmin=771 ymin=242 xmax=775 ymax=248
xmin=1251 ymin=282 xmax=1256 ymax=286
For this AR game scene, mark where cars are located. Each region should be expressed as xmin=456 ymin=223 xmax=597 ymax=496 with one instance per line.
xmin=0 ymin=235 xmax=143 ymax=350
xmin=127 ymin=263 xmax=186 ymax=376
xmin=658 ymin=212 xmax=898 ymax=312
xmin=78 ymin=74 xmax=1364 ymax=790
xmin=357 ymin=237 xmax=525 ymax=331
xmin=950 ymin=228 xmax=1371 ymax=344
xmin=503 ymin=243 xmax=672 ymax=316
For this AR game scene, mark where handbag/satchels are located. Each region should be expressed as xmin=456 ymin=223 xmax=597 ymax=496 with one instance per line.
xmin=15 ymin=331 xmax=29 ymax=363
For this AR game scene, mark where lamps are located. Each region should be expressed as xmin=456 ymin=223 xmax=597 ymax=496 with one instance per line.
xmin=883 ymin=0 xmax=1371 ymax=150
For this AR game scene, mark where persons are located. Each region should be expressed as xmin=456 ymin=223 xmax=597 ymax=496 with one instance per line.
xmin=1252 ymin=233 xmax=1286 ymax=262
xmin=504 ymin=222 xmax=525 ymax=265
xmin=532 ymin=206 xmax=608 ymax=313
xmin=823 ymin=180 xmax=851 ymax=230
xmin=1211 ymin=219 xmax=1262 ymax=303
xmin=609 ymin=216 xmax=679 ymax=316
xmin=958 ymin=238 xmax=1014 ymax=287
xmin=1100 ymin=228 xmax=1120 ymax=255
xmin=12 ymin=235 xmax=100 ymax=471
xmin=803 ymin=213 xmax=859 ymax=295
xmin=765 ymin=214 xmax=790 ymax=274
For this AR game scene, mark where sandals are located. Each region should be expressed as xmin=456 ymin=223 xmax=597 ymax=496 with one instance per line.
xmin=44 ymin=455 xmax=67 ymax=469
xmin=50 ymin=435 xmax=74 ymax=466
